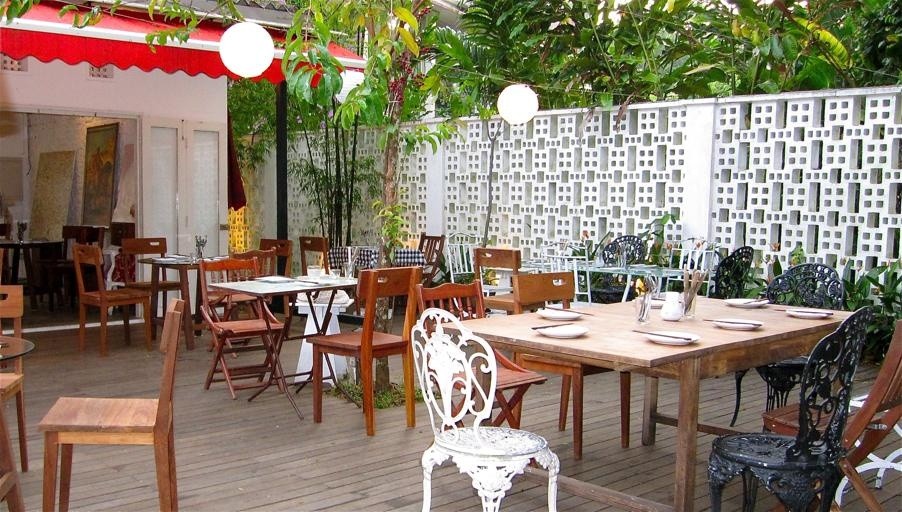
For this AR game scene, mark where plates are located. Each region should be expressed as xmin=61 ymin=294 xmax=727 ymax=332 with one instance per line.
xmin=645 ymin=331 xmax=701 ymax=345
xmin=713 ymin=318 xmax=765 ymax=331
xmin=725 ymin=299 xmax=769 ymax=309
xmin=786 ymin=308 xmax=833 ymax=319
xmin=150 ymin=252 xmax=231 ymax=265
xmin=536 ymin=309 xmax=580 ymax=321
xmin=251 ymin=275 xmax=294 ymax=283
xmin=536 ymin=326 xmax=588 ymax=339
xmin=530 ymin=251 xmax=687 ymax=273
xmin=631 ymin=297 xmax=665 ymax=310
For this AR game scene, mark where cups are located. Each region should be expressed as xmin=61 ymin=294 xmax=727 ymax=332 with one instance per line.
xmin=635 ymin=296 xmax=652 ymax=324
xmin=297 ymin=262 xmax=356 ymax=286
xmin=680 ymin=291 xmax=697 ymax=318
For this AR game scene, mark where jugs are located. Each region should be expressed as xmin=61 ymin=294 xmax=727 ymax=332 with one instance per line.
xmin=659 ymin=291 xmax=686 ymax=322
